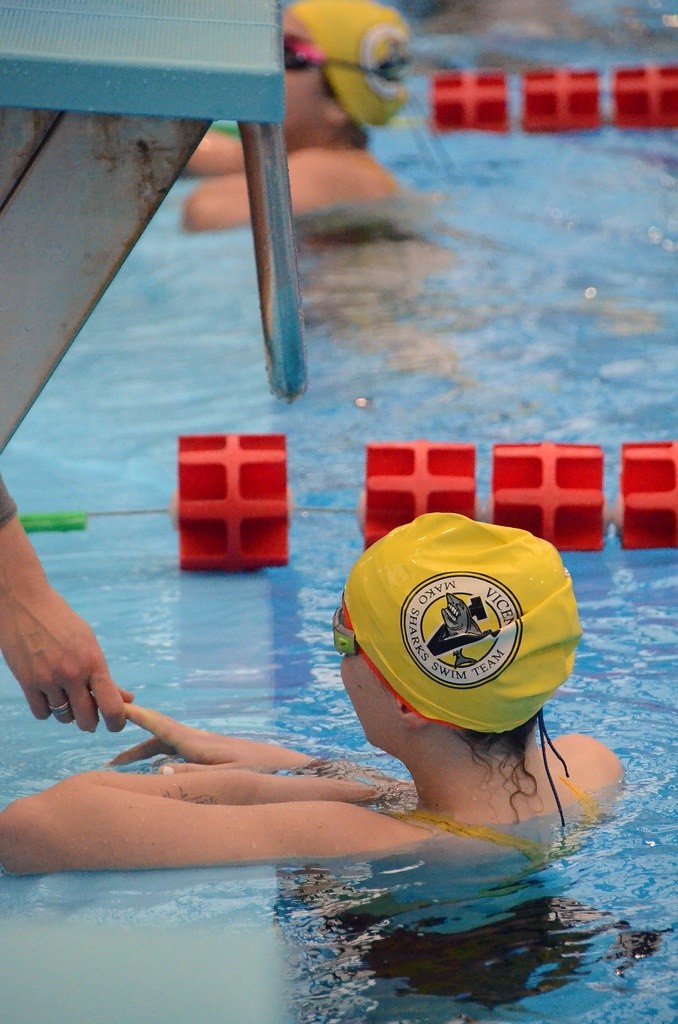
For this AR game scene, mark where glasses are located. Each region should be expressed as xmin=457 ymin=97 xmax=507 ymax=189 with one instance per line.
xmin=333 ymin=607 xmax=359 ymax=654
xmin=282 ymin=36 xmax=326 ymax=70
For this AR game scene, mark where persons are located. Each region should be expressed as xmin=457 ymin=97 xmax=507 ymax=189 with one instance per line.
xmin=0 ymin=476 xmax=133 ymax=732
xmin=0 ymin=513 xmax=625 ymax=872
xmin=175 ymin=0 xmax=411 ymax=229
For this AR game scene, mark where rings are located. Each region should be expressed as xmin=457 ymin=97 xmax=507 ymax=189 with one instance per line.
xmin=48 ymin=702 xmax=69 ymax=715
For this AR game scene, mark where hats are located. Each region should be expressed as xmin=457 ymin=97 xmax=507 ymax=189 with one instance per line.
xmin=286 ymin=0 xmax=412 ymax=137
xmin=338 ymin=511 xmax=583 ymax=733
